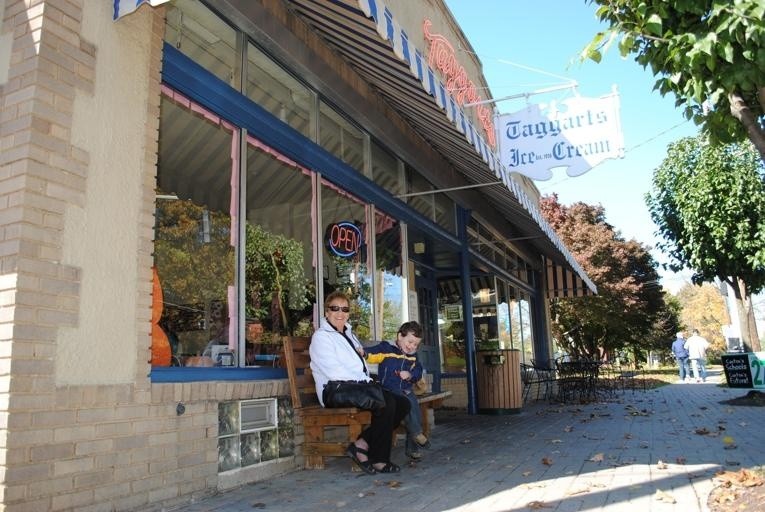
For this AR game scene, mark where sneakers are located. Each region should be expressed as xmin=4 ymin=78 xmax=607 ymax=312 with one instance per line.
xmin=406 ymin=449 xmax=424 ymax=461
xmin=412 ymin=433 xmax=431 ymax=449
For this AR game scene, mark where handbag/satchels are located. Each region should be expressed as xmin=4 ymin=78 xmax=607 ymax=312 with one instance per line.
xmin=411 ymin=375 xmax=427 ymax=396
xmin=323 ymin=381 xmax=386 ymax=410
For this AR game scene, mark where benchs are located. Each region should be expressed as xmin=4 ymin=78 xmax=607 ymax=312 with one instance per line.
xmin=283 ymin=336 xmax=452 ymax=470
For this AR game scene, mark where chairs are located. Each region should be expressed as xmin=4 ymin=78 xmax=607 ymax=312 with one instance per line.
xmin=520 ymin=354 xmax=646 ymax=404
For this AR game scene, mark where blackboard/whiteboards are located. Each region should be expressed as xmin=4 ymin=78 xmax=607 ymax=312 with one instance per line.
xmin=721 ymin=354 xmax=754 ymax=388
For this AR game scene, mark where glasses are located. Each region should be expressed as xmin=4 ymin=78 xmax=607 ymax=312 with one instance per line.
xmin=328 ymin=306 xmax=349 ymax=312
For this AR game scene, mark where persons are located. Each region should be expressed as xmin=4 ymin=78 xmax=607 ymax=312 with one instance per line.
xmin=672 ymin=332 xmax=691 ymax=382
xmin=683 ymin=329 xmax=711 ymax=383
xmin=354 ymin=320 xmax=431 ymax=463
xmin=309 ymin=291 xmax=413 ymax=476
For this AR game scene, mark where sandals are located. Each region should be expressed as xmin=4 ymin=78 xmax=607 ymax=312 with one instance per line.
xmin=345 ymin=442 xmax=376 ymax=474
xmin=373 ymin=463 xmax=400 ymax=473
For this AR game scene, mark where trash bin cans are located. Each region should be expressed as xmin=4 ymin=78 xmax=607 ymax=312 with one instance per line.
xmin=475 ymin=348 xmax=522 ymax=415
